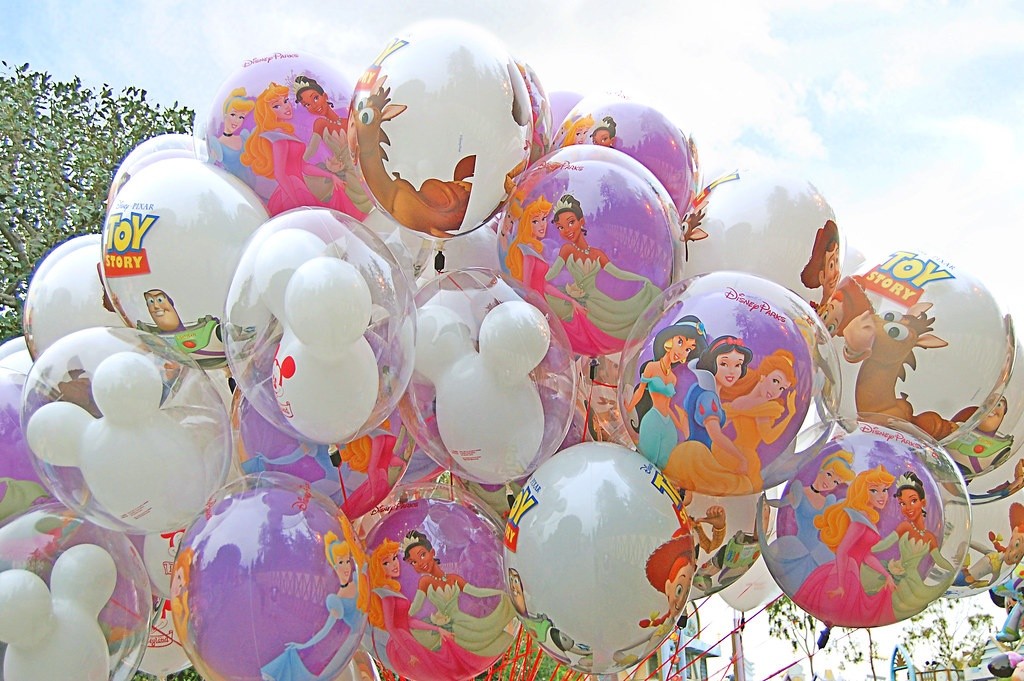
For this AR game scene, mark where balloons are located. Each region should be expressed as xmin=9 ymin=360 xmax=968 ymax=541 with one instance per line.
xmin=0 ymin=19 xmax=1024 ymax=681
